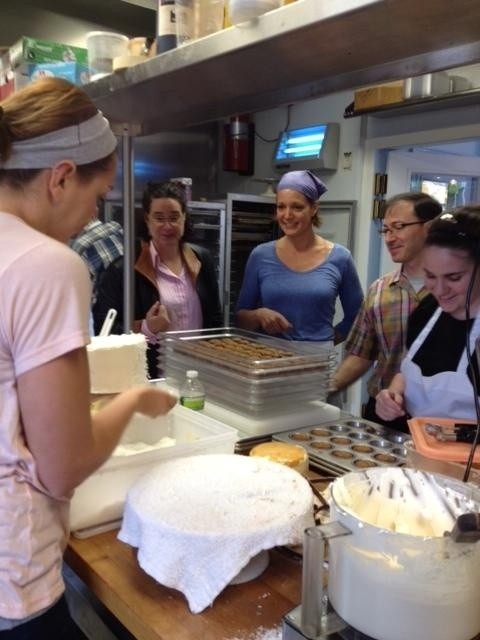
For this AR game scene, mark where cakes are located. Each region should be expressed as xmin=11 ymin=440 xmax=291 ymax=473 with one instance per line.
xmin=249 ymin=441 xmax=310 ymax=480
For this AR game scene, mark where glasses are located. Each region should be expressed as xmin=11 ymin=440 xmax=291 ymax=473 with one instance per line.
xmin=145 ymin=211 xmax=186 ymax=228
xmin=378 ymin=221 xmax=426 ymax=238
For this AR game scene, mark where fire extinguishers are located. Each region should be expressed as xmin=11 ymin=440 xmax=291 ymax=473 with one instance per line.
xmin=228 ymin=114 xmax=251 ymax=172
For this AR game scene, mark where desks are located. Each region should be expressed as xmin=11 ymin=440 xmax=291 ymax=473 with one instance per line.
xmin=50 ymin=525 xmax=324 ymax=640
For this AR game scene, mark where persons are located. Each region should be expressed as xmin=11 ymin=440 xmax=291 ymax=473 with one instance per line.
xmin=232 ymin=170 xmax=365 ymax=360
xmin=69 ymin=212 xmax=129 ymax=308
xmin=361 ymin=208 xmax=480 ymax=432
xmin=1 ymin=75 xmax=179 ymax=640
xmin=93 ymin=178 xmax=224 ymax=381
xmin=330 ymin=193 xmax=443 ymax=432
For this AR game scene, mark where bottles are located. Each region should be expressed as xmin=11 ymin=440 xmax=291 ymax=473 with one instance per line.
xmin=179 ymin=369 xmax=207 ymax=414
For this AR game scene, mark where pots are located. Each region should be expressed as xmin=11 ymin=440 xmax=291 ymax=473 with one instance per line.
xmin=297 ymin=467 xmax=479 ymax=640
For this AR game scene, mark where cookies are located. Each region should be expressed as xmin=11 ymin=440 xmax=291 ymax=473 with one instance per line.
xmin=292 ymin=429 xmax=395 ymax=467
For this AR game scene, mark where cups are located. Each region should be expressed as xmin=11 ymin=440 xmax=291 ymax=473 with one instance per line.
xmin=86 ymin=0 xmax=285 ymax=82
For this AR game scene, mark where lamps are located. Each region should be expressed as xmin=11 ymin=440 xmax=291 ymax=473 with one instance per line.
xmin=271 ymin=121 xmax=342 ymax=176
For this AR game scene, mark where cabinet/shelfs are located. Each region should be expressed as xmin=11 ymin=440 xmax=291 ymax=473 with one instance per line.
xmin=222 ymin=191 xmax=287 ymax=324
xmin=104 ymin=197 xmax=225 ymax=326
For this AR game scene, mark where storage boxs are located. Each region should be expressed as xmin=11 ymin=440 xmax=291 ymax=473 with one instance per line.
xmin=355 ymin=82 xmax=405 ymax=112
xmin=46 ymin=391 xmax=239 ymax=534
xmin=0 ymin=34 xmax=88 ymax=98
